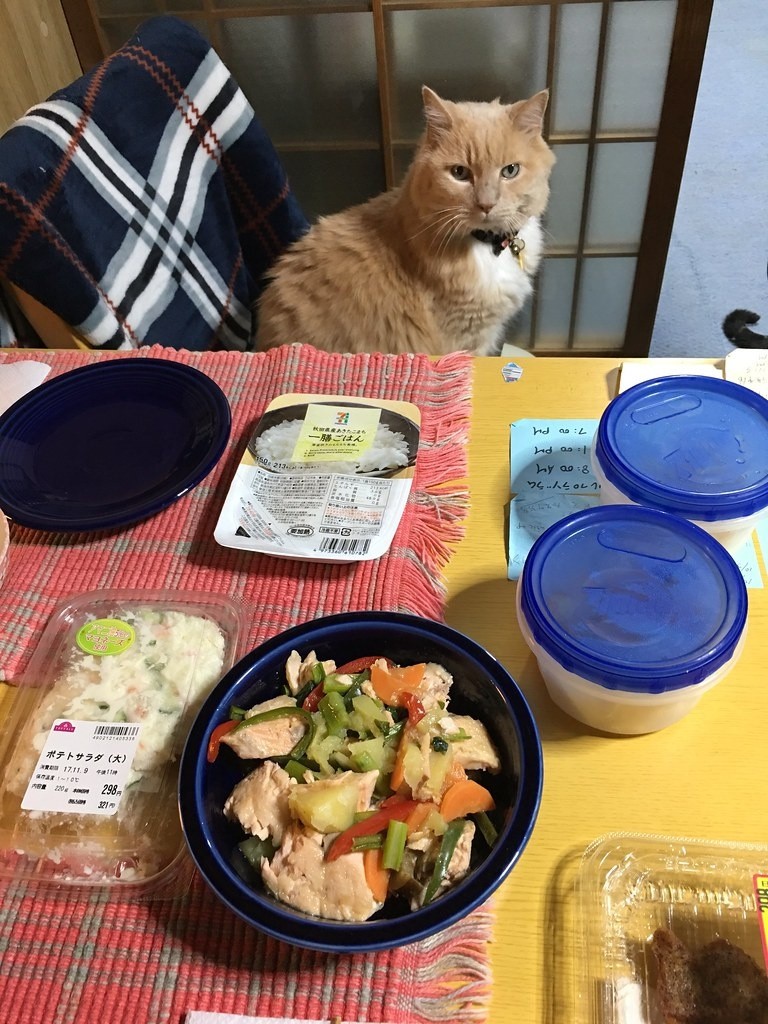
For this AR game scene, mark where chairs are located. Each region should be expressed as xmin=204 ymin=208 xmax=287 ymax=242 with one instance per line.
xmin=0 ymin=12 xmax=317 ymax=354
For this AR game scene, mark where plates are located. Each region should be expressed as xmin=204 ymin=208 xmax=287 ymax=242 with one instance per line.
xmin=1 ymin=357 xmax=230 ymax=533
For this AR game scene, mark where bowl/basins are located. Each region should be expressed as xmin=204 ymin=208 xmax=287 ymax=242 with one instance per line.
xmin=253 ymin=400 xmax=421 ymax=482
xmin=177 ymin=612 xmax=548 ymax=952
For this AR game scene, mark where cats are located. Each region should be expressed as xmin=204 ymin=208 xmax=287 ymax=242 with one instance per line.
xmin=246 ymin=85 xmax=556 ymax=358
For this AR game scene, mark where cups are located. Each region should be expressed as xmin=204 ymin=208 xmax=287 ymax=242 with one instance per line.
xmin=589 ymin=376 xmax=768 ymax=560
xmin=514 ymin=507 xmax=747 ymax=740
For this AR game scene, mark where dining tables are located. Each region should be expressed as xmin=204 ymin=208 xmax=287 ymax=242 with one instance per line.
xmin=1 ymin=344 xmax=768 ymax=1024
